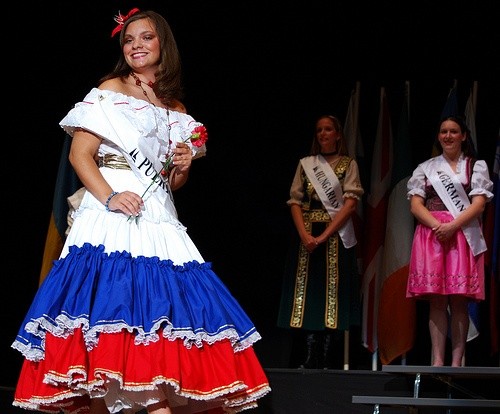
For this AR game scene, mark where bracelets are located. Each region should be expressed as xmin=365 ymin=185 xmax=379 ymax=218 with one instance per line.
xmin=104 ymin=191 xmax=120 ymax=213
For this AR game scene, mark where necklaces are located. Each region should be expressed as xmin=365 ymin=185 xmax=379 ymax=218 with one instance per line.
xmin=130 ymin=69 xmax=172 ymax=160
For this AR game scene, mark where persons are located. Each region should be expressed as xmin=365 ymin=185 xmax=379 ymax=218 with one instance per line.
xmin=401 ymin=116 xmax=495 ymax=369
xmin=284 ymin=112 xmax=367 ymax=373
xmin=9 ymin=7 xmax=274 ymax=414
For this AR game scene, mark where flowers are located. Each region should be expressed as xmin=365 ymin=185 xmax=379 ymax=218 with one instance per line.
xmin=127 ymin=126 xmax=208 ymax=225
xmin=111 ymin=8 xmax=140 ymax=38
